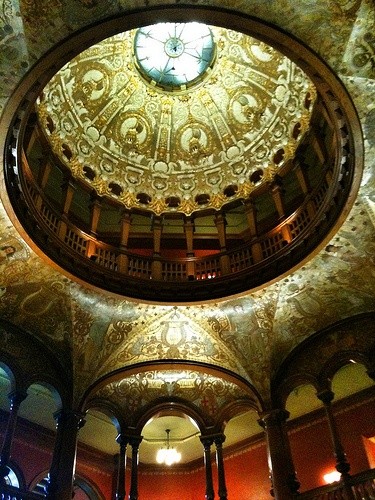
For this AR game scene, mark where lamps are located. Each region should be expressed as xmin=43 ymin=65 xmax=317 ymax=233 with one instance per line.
xmin=155 ymin=429 xmax=182 ymax=465
